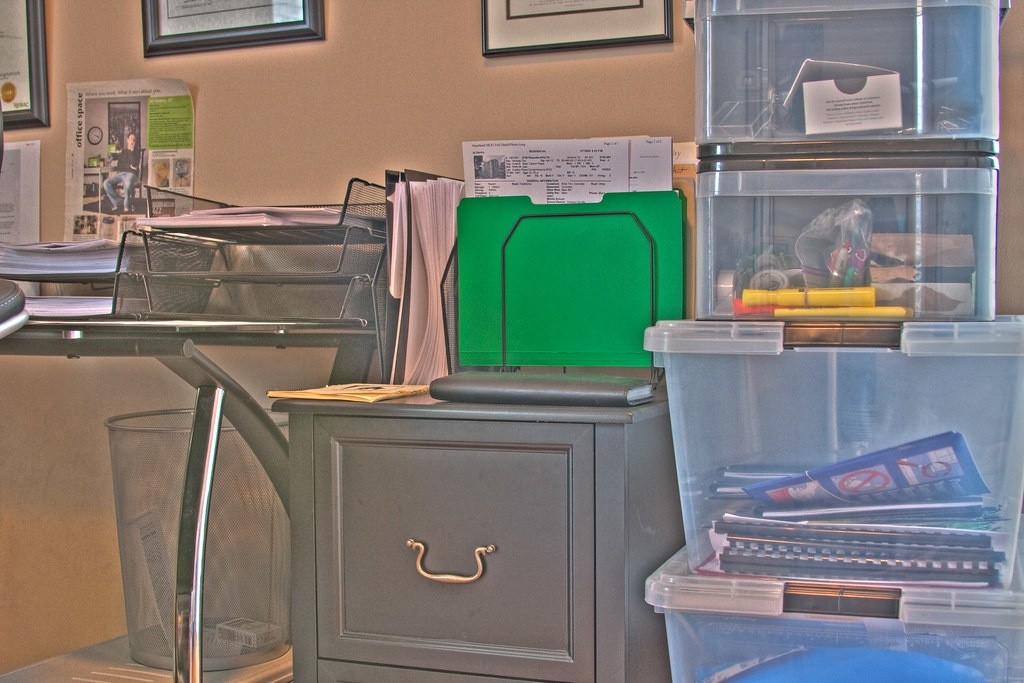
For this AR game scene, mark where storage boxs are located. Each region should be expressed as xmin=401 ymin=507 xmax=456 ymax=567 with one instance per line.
xmin=644 ymin=1 xmax=1024 ymax=683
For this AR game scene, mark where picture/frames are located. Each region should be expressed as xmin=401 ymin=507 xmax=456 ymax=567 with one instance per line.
xmin=481 ymin=0 xmax=674 ymax=57
xmin=141 ymin=0 xmax=326 ymax=58
xmin=1 ymin=0 xmax=51 ymax=131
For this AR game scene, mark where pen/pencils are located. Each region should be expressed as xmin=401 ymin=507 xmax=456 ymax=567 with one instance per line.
xmin=828 ymin=241 xmax=866 ymax=289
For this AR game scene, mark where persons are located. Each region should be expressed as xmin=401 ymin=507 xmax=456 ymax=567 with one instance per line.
xmin=101 ymin=133 xmax=141 ymax=215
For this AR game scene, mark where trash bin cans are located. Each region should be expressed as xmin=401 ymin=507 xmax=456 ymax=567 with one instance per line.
xmin=103 ymin=406 xmax=290 ymax=674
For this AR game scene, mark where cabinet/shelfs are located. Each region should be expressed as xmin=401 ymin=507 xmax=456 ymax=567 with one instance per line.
xmin=271 ymin=390 xmax=685 ymax=683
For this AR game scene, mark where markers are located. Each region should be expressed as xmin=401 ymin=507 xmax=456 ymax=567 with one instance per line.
xmin=733 ymin=287 xmax=907 ymax=319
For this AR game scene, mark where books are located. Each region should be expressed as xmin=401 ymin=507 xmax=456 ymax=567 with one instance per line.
xmin=710 ymin=430 xmax=1005 ymax=589
xmin=864 ymin=231 xmax=976 ymax=318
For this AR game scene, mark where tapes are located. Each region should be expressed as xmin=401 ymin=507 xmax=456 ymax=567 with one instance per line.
xmin=717 ymin=269 xmax=742 ymax=305
xmin=748 ymin=267 xmax=789 ymax=292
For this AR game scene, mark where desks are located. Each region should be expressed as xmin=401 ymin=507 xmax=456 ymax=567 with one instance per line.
xmin=0 ymin=320 xmax=378 ymax=683
